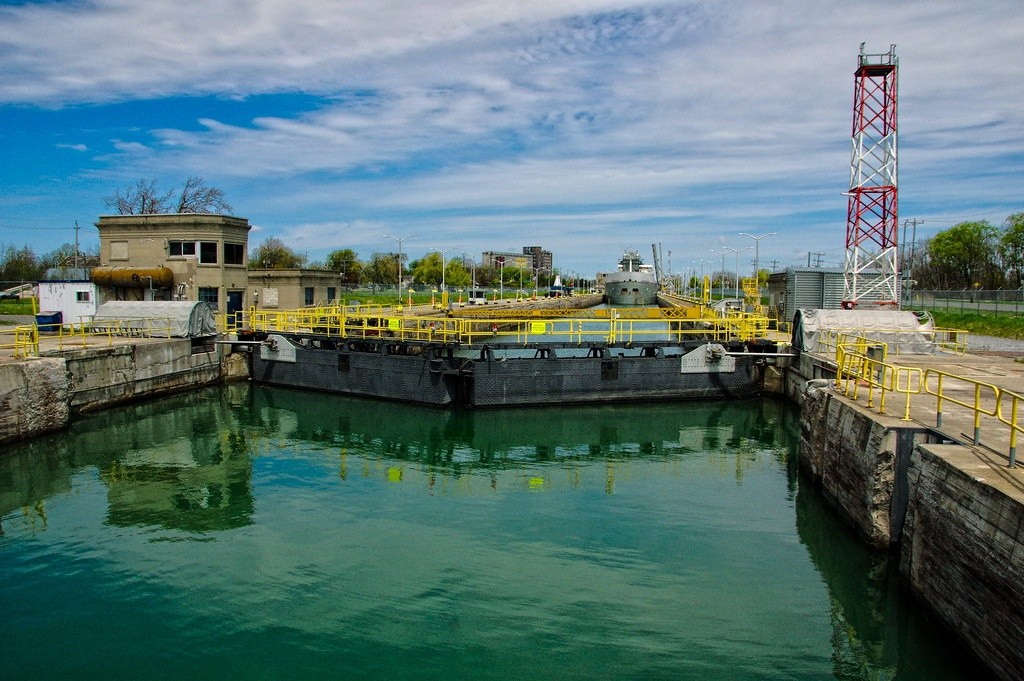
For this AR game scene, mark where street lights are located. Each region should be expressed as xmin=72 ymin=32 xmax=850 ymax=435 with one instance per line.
xmin=673 ymin=259 xmax=724 ymax=303
xmin=384 ymin=234 xmax=415 ymax=303
xmin=430 ymin=247 xmax=457 ymax=291
xmin=723 ymin=246 xmax=754 ymax=298
xmin=709 ymin=250 xmax=735 ymax=300
xmin=491 ymin=259 xmax=512 ymax=302
xmin=512 ymin=261 xmax=530 ymax=298
xmin=462 ymin=251 xmax=488 ymax=299
xmin=528 ymin=266 xmax=546 ymax=298
xmin=739 ymin=232 xmax=776 ymax=276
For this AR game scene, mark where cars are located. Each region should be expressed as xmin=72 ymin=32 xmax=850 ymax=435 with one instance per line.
xmin=0 ymin=293 xmax=19 ymax=300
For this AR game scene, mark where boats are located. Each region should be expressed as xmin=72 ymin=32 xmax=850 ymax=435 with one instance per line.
xmin=604 ymin=246 xmax=657 ymax=305
xmin=544 ymin=273 xmax=574 ymax=298
